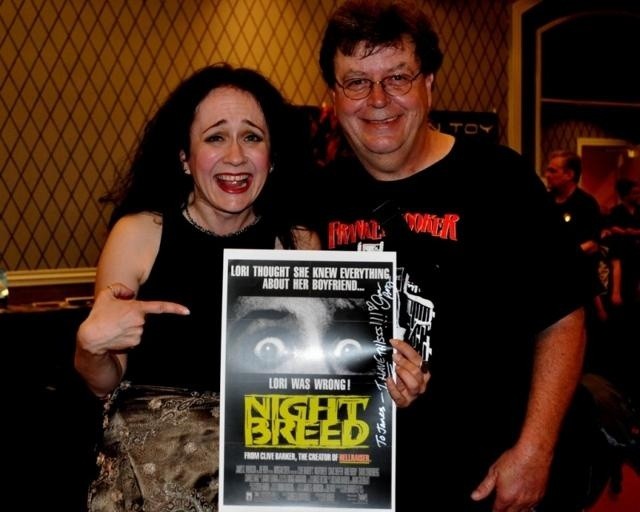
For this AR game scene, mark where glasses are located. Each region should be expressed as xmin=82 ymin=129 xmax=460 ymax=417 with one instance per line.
xmin=333 ymin=69 xmax=421 ymax=101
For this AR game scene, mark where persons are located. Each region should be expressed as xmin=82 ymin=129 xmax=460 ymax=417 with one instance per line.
xmin=229 ymin=290 xmax=381 ymax=375
xmin=75 ymin=60 xmax=435 ymax=512
xmin=536 ymin=147 xmax=640 ymax=414
xmin=278 ymin=1 xmax=593 ymax=511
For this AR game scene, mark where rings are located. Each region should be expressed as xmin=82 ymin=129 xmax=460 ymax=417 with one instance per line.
xmin=419 ymin=360 xmax=428 ymax=373
